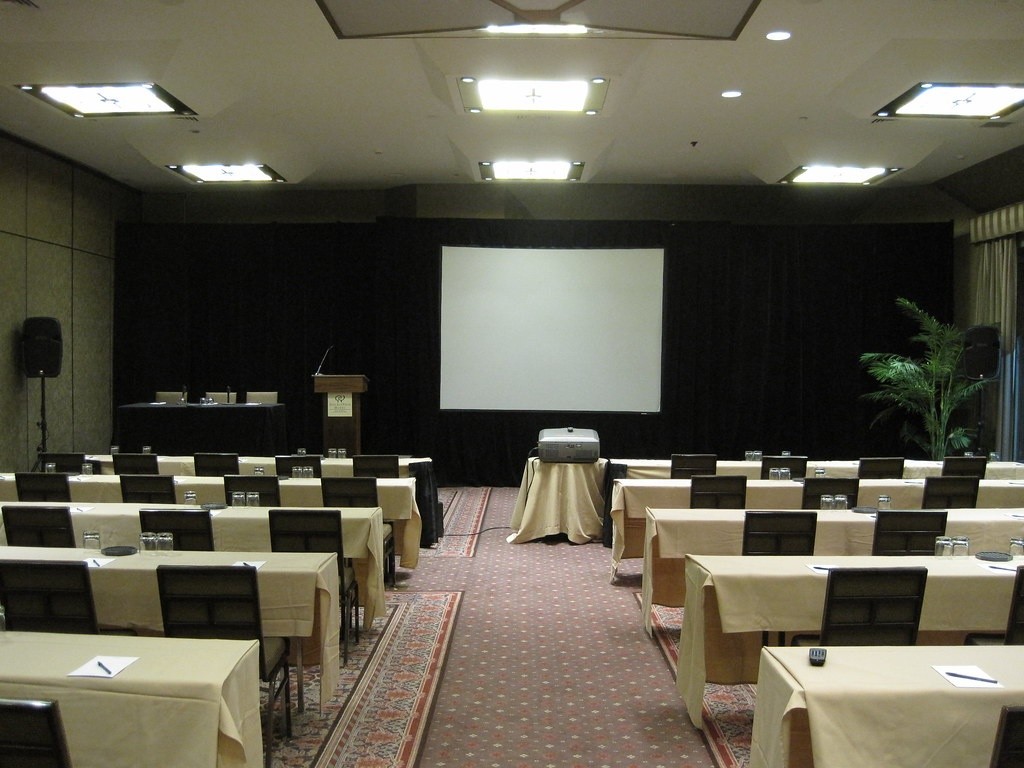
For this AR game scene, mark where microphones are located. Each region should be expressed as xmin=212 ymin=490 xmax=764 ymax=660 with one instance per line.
xmin=177 ymin=385 xmax=188 ymax=404
xmin=222 ymin=385 xmax=234 ymax=405
xmin=315 ymin=345 xmax=334 ymax=376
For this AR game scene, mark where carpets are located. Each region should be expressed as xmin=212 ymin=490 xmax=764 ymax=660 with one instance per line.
xmin=417 ymin=485 xmax=493 ymax=559
xmin=633 ymin=590 xmax=757 ymax=768
xmin=261 ymin=588 xmax=465 ymax=768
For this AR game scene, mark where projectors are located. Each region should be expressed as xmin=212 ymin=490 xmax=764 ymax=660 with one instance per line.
xmin=538 ymin=425 xmax=600 ymax=463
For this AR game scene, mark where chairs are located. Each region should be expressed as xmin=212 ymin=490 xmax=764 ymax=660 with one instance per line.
xmin=690 ymin=474 xmax=749 ymax=510
xmin=669 ymin=452 xmax=719 ymax=478
xmin=871 ymin=507 xmax=948 ymax=556
xmin=962 ymin=561 xmax=1024 ymax=646
xmin=0 ymin=557 xmax=102 ymax=635
xmin=223 ymin=474 xmax=282 ymax=508
xmin=801 ymin=476 xmax=862 ymax=509
xmin=193 ymin=452 xmax=241 ymax=476
xmin=267 ymin=508 xmax=361 ymax=666
xmin=1 ymin=505 xmax=79 ymax=549
xmin=788 ymin=563 xmax=929 ymax=647
xmin=245 ymin=390 xmax=279 ymax=403
xmin=274 ymin=454 xmax=323 ymax=478
xmin=137 ymin=508 xmax=215 ymax=552
xmin=942 ymin=454 xmax=988 ymax=479
xmin=988 ymin=703 xmax=1024 ymax=768
xmin=858 ymin=457 xmax=906 ymax=479
xmin=155 ymin=564 xmax=296 ymax=768
xmin=0 ymin=696 xmax=72 ymax=768
xmin=351 ymin=452 xmax=399 ymax=477
xmin=111 ymin=452 xmax=161 ymax=473
xmin=119 ymin=473 xmax=178 ymax=504
xmin=319 ymin=475 xmax=397 ymax=590
xmin=15 ymin=472 xmax=72 ymax=502
xmin=757 ymin=455 xmax=809 ymax=478
xmin=39 ymin=451 xmax=87 ymax=473
xmin=742 ymin=508 xmax=818 ymax=557
xmin=205 ymin=391 xmax=237 ymax=403
xmin=154 ymin=390 xmax=189 ymax=402
xmin=920 ymin=474 xmax=980 ymax=509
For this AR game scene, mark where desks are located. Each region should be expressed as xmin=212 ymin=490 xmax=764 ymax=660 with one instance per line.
xmin=0 ymin=401 xmax=1024 ymax=768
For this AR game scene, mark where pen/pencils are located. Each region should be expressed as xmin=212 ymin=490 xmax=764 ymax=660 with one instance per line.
xmin=946 ymin=672 xmax=998 ymax=683
xmin=93 ymin=559 xmax=100 ymax=566
xmin=905 ymin=482 xmax=923 ymax=484
xmin=1012 ymin=515 xmax=1024 ymax=517
xmin=97 ymin=661 xmax=111 ymax=674
xmin=871 ymin=516 xmax=876 ymax=518
xmin=243 ymin=562 xmax=249 ymax=566
xmin=989 ymin=566 xmax=1017 ymax=571
xmin=813 ymin=566 xmax=828 ymax=570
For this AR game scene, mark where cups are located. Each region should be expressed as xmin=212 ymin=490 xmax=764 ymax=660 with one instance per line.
xmin=745 ymin=450 xmax=762 ymax=460
xmin=143 ymin=446 xmax=152 ymax=454
xmin=82 ymin=463 xmax=92 ymax=475
xmin=820 ymin=495 xmax=848 ymax=509
xmin=298 ymin=448 xmax=346 ymax=458
xmin=964 ymin=452 xmax=973 ymax=457
xmin=1010 ymin=536 xmax=1024 ymax=556
xmin=815 ymin=467 xmax=825 ymax=478
xmin=769 ymin=468 xmax=790 ymax=480
xmin=253 ymin=466 xmax=313 ymax=477
xmin=111 ymin=445 xmax=119 ymax=454
xmin=184 ymin=491 xmax=259 ymax=506
xmin=878 ymin=495 xmax=891 ymax=510
xmin=180 ymin=397 xmax=214 ymax=407
xmin=82 ymin=531 xmax=173 ymax=560
xmin=782 ymin=451 xmax=791 ymax=456
xmin=0 ymin=604 xmax=6 ymax=666
xmin=934 ymin=536 xmax=968 ymax=556
xmin=989 ymin=452 xmax=1000 ymax=462
xmin=45 ymin=463 xmax=56 ymax=472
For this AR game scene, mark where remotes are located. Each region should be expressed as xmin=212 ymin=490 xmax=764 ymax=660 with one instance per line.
xmin=809 ymin=647 xmax=827 ymax=666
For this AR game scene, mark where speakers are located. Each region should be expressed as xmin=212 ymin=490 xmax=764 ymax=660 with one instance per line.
xmin=964 ymin=327 xmax=1000 ymax=381
xmin=22 ymin=317 xmax=63 ymax=378
